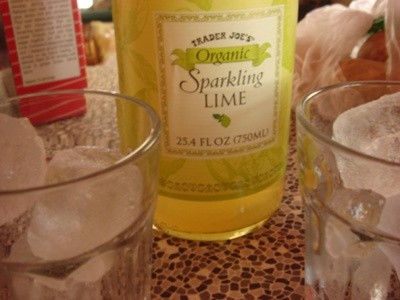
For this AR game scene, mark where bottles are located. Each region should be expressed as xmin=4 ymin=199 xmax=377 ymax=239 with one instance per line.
xmin=111 ymin=0 xmax=299 ymax=240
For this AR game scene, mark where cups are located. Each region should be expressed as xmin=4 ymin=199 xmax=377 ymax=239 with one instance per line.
xmin=296 ymin=80 xmax=400 ymax=300
xmin=0 ymin=89 xmax=163 ymax=299
